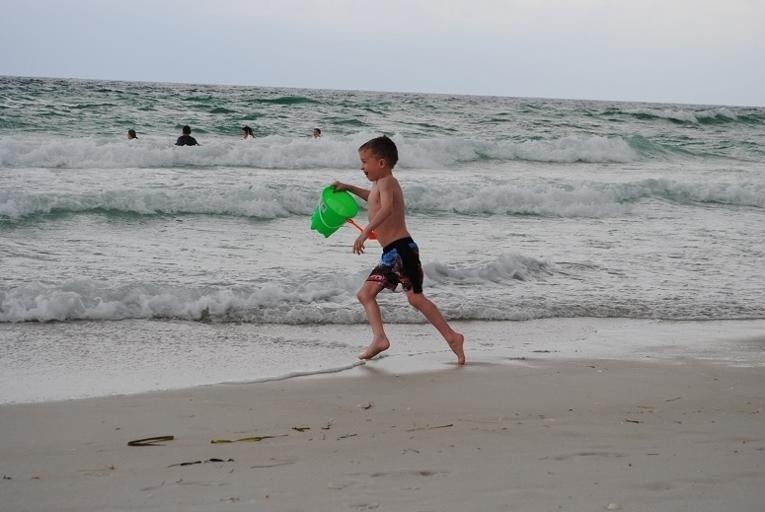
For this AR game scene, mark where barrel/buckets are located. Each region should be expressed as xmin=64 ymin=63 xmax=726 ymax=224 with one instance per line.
xmin=310 ymin=184 xmax=358 ymax=238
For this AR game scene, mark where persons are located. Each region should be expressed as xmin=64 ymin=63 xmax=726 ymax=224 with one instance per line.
xmin=127 ymin=128 xmax=137 ymax=139
xmin=241 ymin=125 xmax=255 ymax=140
xmin=174 ymin=124 xmax=198 ymax=146
xmin=331 ymin=134 xmax=465 ymax=366
xmin=313 ymin=127 xmax=320 ymax=138
